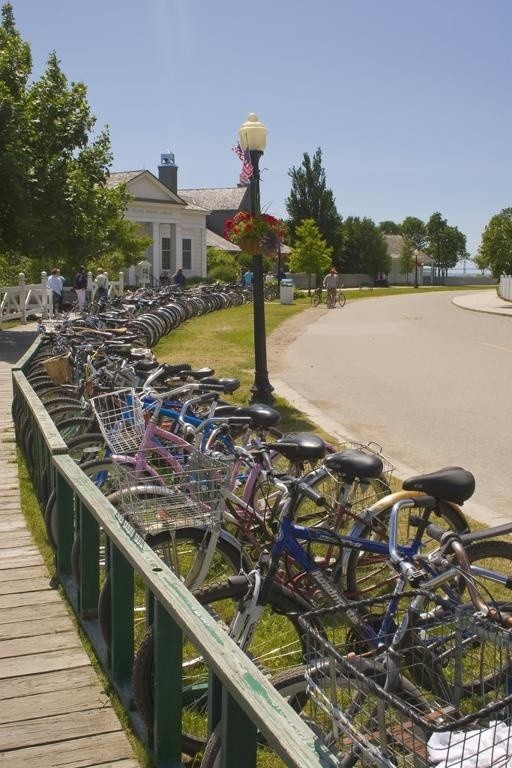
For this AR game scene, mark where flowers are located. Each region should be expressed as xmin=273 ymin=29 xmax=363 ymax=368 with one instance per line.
xmin=222 ymin=212 xmax=287 ymax=244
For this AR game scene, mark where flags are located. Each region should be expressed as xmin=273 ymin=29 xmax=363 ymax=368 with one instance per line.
xmin=233 ymin=142 xmax=245 ymax=163
xmin=238 ymin=145 xmax=254 ymax=189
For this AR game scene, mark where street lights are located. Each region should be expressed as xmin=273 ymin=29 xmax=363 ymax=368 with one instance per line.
xmin=237 ymin=113 xmax=275 ymax=406
xmin=414 ymin=248 xmax=419 ymax=288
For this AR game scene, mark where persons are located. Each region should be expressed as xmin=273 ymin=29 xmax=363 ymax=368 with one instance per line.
xmin=171 ymin=268 xmax=186 ymax=287
xmin=323 ymin=267 xmax=339 ymax=309
xmin=277 ymin=267 xmax=286 ymax=281
xmin=57 ymin=268 xmax=67 ymax=312
xmin=235 ymin=269 xmax=243 ymax=286
xmin=47 ymin=268 xmax=64 ymax=315
xmin=91 ymin=267 xmax=110 ymax=303
xmin=240 ymin=268 xmax=247 ymax=290
xmin=73 ymin=265 xmax=89 ymax=310
xmin=242 ymin=268 xmax=253 ymax=289
xmin=158 ymin=270 xmax=172 ymax=286
xmin=264 ymin=271 xmax=275 ymax=281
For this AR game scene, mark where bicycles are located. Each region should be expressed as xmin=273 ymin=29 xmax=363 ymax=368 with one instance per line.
xmin=37 ymin=280 xmax=477 ymax=682
xmin=296 ymin=524 xmax=512 ymax=768
xmin=131 ymin=439 xmax=512 ymax=760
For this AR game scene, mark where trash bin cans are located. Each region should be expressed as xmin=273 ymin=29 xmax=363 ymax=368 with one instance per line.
xmin=279 ymin=278 xmax=294 ymax=305
